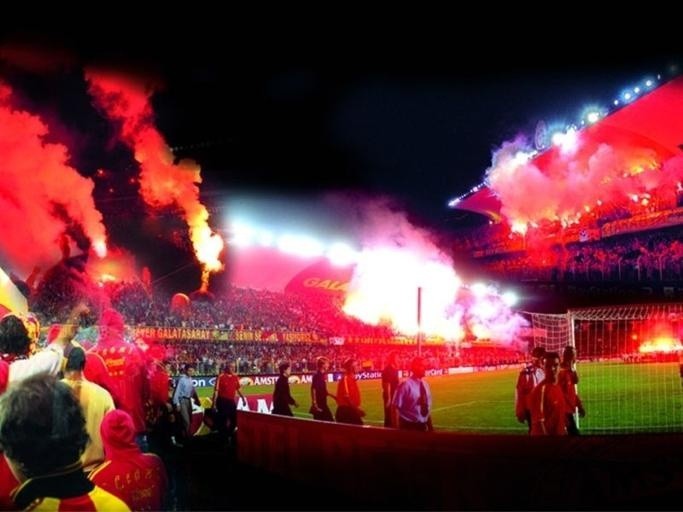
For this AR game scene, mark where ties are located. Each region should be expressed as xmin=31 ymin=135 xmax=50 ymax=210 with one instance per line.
xmin=419 ymin=380 xmax=429 ymax=418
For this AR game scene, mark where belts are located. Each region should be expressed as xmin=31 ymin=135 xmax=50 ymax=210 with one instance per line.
xmin=182 ymin=395 xmax=190 ymax=400
